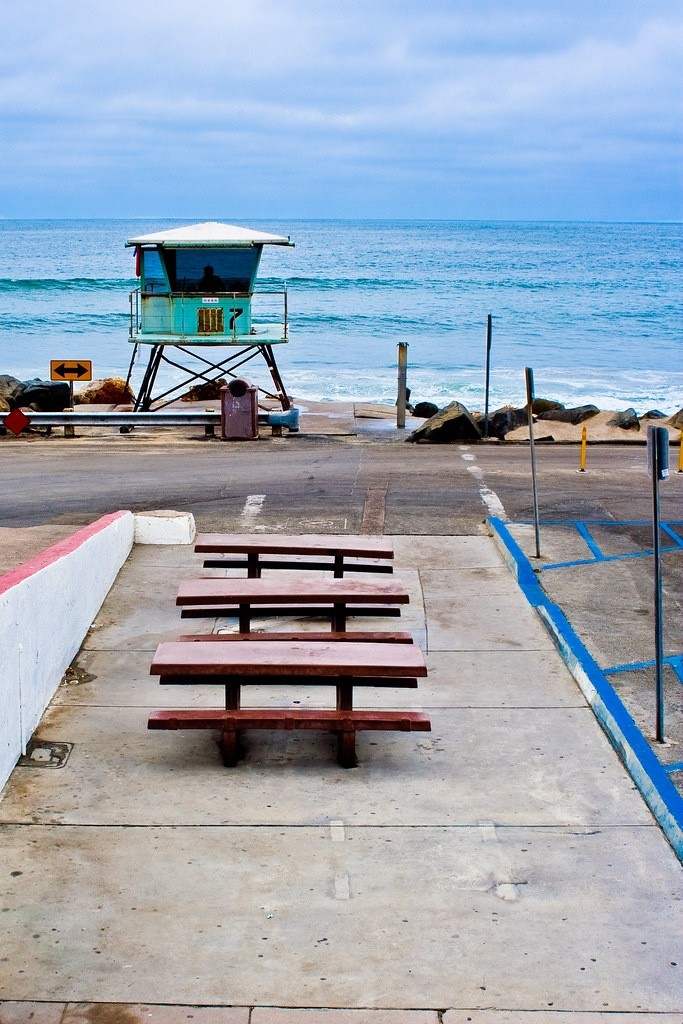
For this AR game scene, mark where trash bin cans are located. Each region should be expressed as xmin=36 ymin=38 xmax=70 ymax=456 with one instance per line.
xmin=221 ymin=378 xmax=260 ymax=439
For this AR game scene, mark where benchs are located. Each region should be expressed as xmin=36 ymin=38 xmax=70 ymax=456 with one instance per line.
xmin=158 ymin=673 xmax=419 ymax=688
xmin=147 ymin=710 xmax=432 ymax=733
xmin=203 ymin=556 xmax=393 ymax=574
xmin=179 ymin=632 xmax=413 ymax=645
xmin=181 ymin=602 xmax=401 ymax=617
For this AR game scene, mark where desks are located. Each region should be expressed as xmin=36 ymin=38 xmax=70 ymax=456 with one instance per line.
xmin=175 ymin=579 xmax=409 ymax=631
xmin=194 ymin=535 xmax=394 ymax=579
xmin=150 ymin=642 xmax=428 ymax=768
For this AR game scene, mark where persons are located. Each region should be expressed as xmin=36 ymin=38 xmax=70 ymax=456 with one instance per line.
xmin=199 ymin=266 xmax=222 ymax=293
